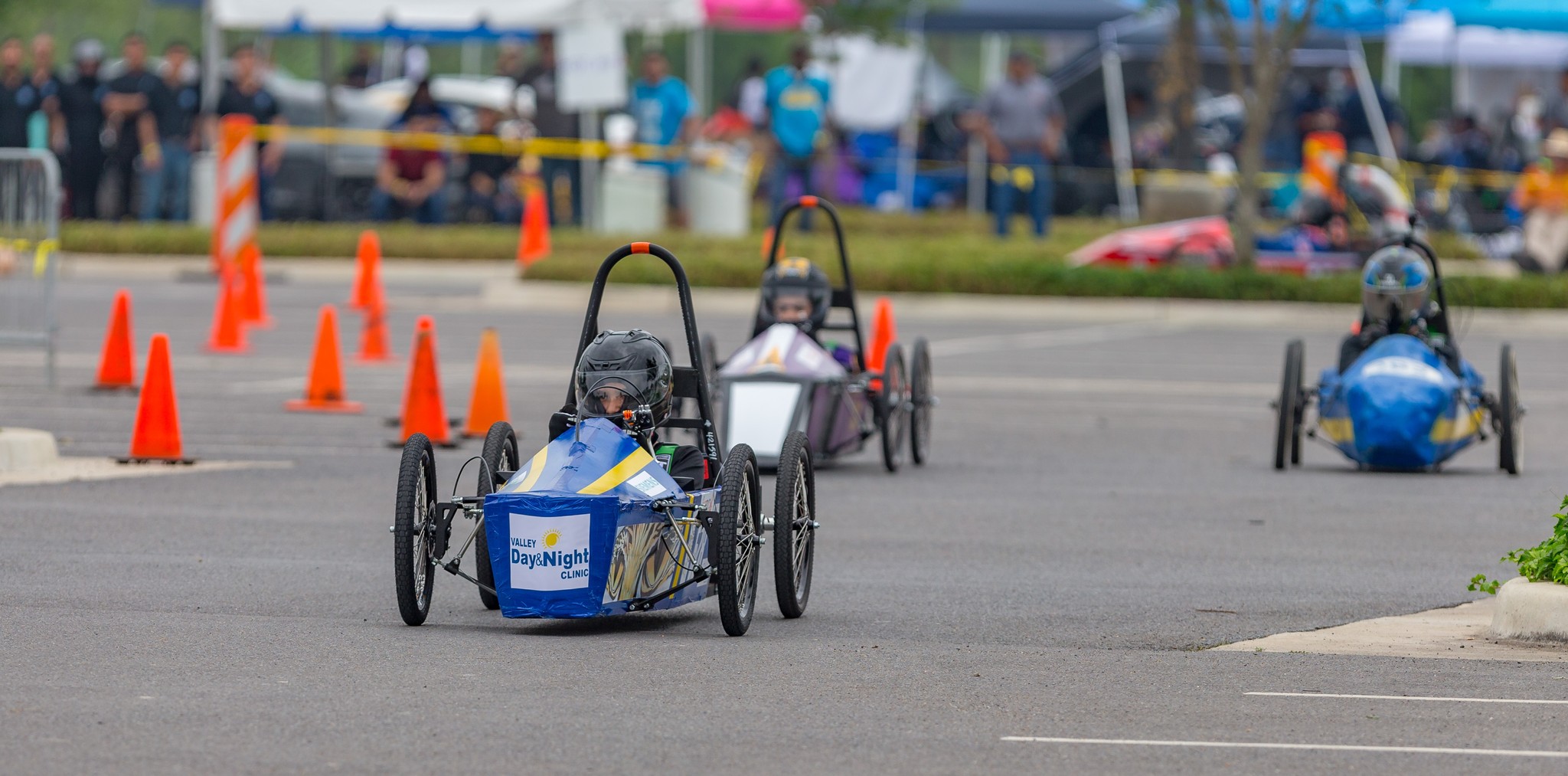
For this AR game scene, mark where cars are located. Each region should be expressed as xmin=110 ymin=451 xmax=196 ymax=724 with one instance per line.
xmin=56 ymin=53 xmax=634 ymax=223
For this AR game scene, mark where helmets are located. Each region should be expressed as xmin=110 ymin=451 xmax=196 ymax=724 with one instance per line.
xmin=757 ymin=257 xmax=832 ymax=335
xmin=574 ymin=329 xmax=673 ymax=432
xmin=1360 ymin=244 xmax=1435 ymax=326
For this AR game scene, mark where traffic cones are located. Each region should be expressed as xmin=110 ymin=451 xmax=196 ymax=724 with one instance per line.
xmin=866 ymin=304 xmax=911 ymax=393
xmin=350 ymin=230 xmax=398 ymax=364
xmin=83 ymin=288 xmax=142 ymax=395
xmin=380 ymin=314 xmax=521 ymax=452
xmin=112 ymin=334 xmax=193 ymax=466
xmin=205 ymin=241 xmax=273 ymax=353
xmin=286 ymin=305 xmax=362 ymax=411
xmin=515 ymin=180 xmax=550 ymax=268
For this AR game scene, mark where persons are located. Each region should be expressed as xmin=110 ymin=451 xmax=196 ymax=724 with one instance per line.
xmin=974 ymin=45 xmax=1066 ymax=239
xmin=0 ymin=32 xmax=281 ymax=223
xmin=344 ymin=33 xmax=700 ymax=229
xmin=1070 ymin=63 xmax=1568 ymax=267
xmin=739 ymin=39 xmax=831 ymax=233
xmin=550 ymin=327 xmax=706 ymax=496
xmin=1336 ymin=245 xmax=1463 ymax=387
xmin=759 ymin=256 xmax=850 ymax=372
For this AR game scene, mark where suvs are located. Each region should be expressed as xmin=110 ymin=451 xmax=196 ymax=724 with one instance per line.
xmin=918 ymin=30 xmax=1363 ymax=174
